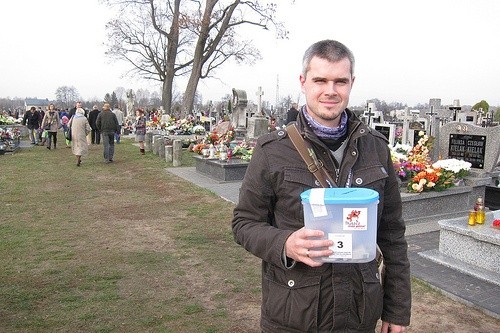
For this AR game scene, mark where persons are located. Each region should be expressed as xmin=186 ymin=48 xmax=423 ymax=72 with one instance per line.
xmin=2 ymin=101 xmax=158 ymax=167
xmin=231 ymin=40 xmax=412 ymax=333
xmin=269 ymin=120 xmax=277 ymax=133
xmin=394 ymin=126 xmax=402 ymax=147
xmin=286 ymin=103 xmax=299 ymax=125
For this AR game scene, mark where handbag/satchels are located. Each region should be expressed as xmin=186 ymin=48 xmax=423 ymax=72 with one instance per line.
xmin=44 ymin=123 xmax=50 ymax=130
xmin=41 ymin=131 xmax=48 ymax=137
xmin=67 ymin=125 xmax=71 ymax=141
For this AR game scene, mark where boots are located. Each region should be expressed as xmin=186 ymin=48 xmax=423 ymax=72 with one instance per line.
xmin=138 ymin=147 xmax=145 ymax=155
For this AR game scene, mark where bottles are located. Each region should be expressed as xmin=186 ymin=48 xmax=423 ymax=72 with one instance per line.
xmin=469 ymin=195 xmax=485 ymax=225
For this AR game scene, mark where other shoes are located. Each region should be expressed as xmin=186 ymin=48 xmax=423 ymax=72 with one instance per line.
xmin=104 ymin=159 xmax=113 ymax=163
xmin=31 ymin=141 xmax=38 ymax=145
xmin=53 ymin=145 xmax=56 ymax=149
xmin=46 ymin=146 xmax=51 ymax=150
xmin=76 ymin=159 xmax=81 ymax=166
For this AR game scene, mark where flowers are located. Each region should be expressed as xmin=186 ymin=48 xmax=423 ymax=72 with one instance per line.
xmin=192 ymin=123 xmax=254 ymax=162
xmin=145 ymin=106 xmax=192 ymax=135
xmin=389 ymin=130 xmax=473 ymax=193
xmin=200 ymin=110 xmax=215 ymax=123
xmin=0 ymin=115 xmax=23 ymax=149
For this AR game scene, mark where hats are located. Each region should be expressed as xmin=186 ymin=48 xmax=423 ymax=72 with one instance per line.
xmin=102 ymin=104 xmax=110 ymax=110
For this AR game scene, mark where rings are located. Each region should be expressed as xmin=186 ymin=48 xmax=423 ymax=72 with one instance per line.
xmin=307 ymin=249 xmax=311 ymax=257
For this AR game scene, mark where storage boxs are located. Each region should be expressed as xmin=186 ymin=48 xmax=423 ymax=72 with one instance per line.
xmin=300 ymin=187 xmax=380 ymax=263
